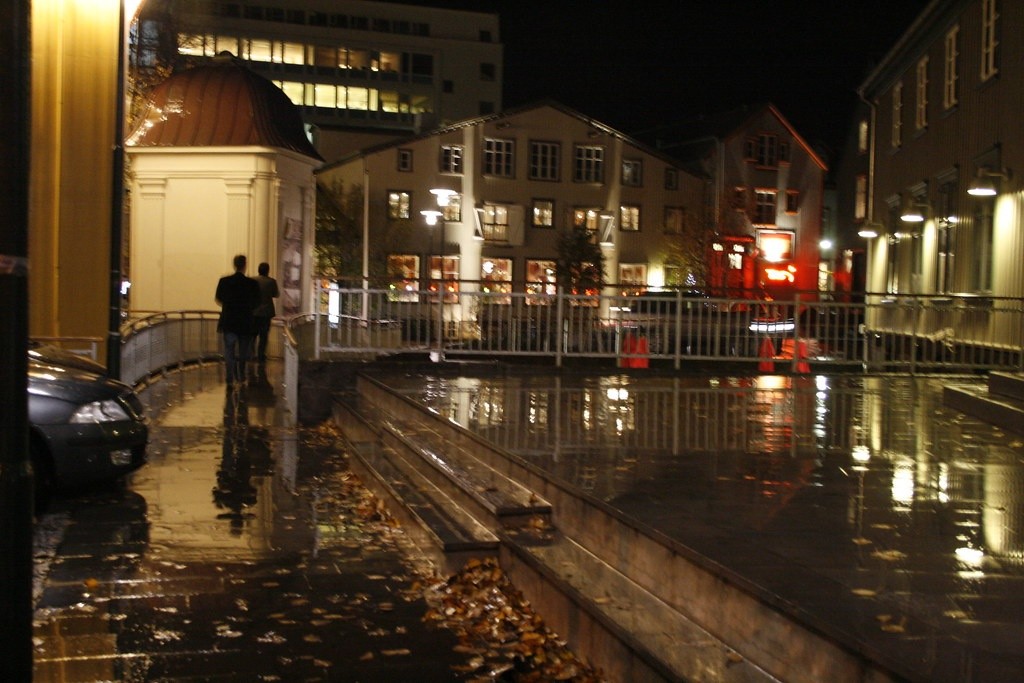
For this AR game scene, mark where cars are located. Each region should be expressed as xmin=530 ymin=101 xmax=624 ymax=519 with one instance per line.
xmin=27 ymin=337 xmax=151 ymax=495
xmin=601 ymin=291 xmax=777 ymax=354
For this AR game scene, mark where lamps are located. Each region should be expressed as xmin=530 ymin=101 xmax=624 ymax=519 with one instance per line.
xmin=966 ymin=166 xmax=1012 ymax=196
xmin=901 ymin=199 xmax=933 ymax=224
xmin=857 ymin=218 xmax=886 ymax=238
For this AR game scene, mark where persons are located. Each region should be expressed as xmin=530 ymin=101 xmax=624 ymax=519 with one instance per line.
xmin=215 ymin=255 xmax=261 ymax=382
xmin=248 ymin=262 xmax=281 ymax=362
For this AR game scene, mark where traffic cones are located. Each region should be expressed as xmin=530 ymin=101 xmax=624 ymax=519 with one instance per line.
xmin=792 ymin=336 xmax=810 ymax=375
xmin=615 ymin=332 xmax=631 ymax=369
xmin=632 ymin=334 xmax=650 ymax=368
xmin=757 ymin=334 xmax=775 ymax=372
